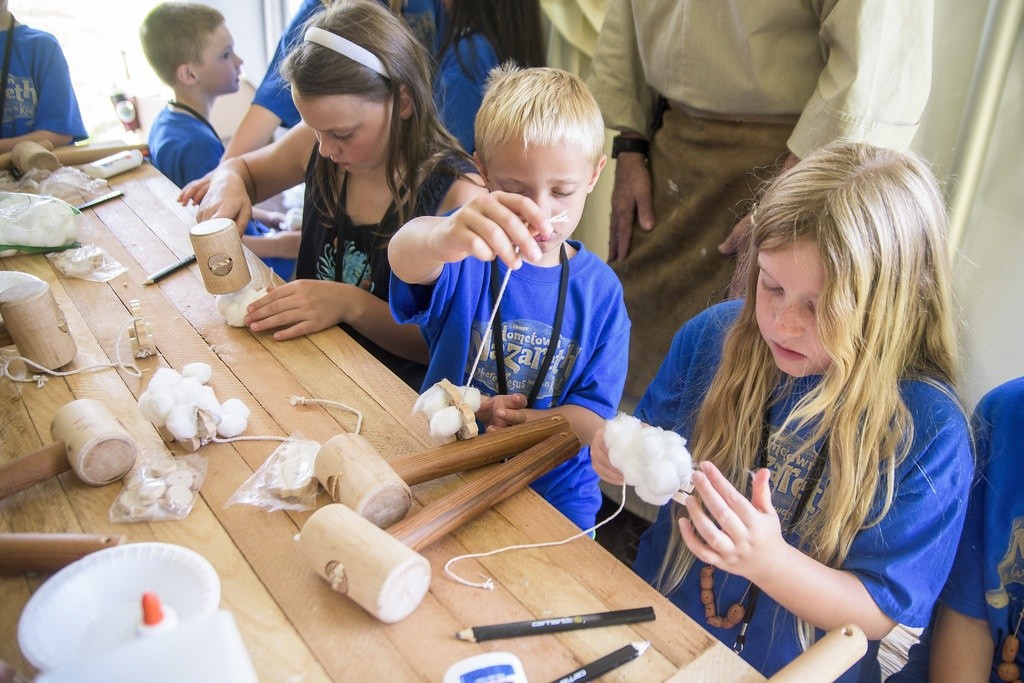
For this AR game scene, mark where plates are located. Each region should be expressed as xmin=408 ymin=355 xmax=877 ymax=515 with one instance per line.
xmin=14 ymin=540 xmax=222 ymax=672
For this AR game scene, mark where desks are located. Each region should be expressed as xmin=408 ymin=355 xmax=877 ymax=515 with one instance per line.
xmin=1 ymin=139 xmax=766 ymax=683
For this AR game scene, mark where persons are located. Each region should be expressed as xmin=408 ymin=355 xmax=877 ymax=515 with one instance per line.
xmin=193 ymin=0 xmax=490 ymax=397
xmin=883 ymin=378 xmax=1024 ymax=683
xmin=387 ymin=59 xmax=631 ymax=540
xmin=531 ymin=0 xmax=937 ymax=568
xmin=589 ymin=136 xmax=977 ymax=683
xmin=140 ymin=0 xmax=304 ymax=284
xmin=0 ymin=0 xmax=88 ymax=152
xmin=178 ymin=1 xmax=541 ymax=209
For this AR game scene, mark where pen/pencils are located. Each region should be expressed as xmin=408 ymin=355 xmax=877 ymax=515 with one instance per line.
xmin=142 ymin=254 xmax=196 ymax=285
xmin=77 ymin=191 xmax=124 ymax=211
xmin=550 ymin=642 xmax=651 ymax=683
xmin=455 ymin=606 xmax=657 ymax=644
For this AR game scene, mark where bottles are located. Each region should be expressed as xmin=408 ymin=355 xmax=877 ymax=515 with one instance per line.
xmin=443 ymin=650 xmax=528 ymax=682
xmin=84 ymin=147 xmax=148 ymax=179
xmin=133 ymin=591 xmax=180 ymax=642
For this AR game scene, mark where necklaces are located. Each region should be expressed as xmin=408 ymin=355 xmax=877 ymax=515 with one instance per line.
xmin=700 ymin=373 xmax=830 ymax=628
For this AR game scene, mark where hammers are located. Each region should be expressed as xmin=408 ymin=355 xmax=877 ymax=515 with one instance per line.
xmin=313 ymin=414 xmax=571 ymax=529
xmin=190 ymin=217 xmax=252 ymax=295
xmin=11 ymin=140 xmax=150 ymax=175
xmin=0 ymin=281 xmax=77 ymax=373
xmin=298 ymin=429 xmax=583 ymax=624
xmin=0 ymin=398 xmax=137 ymax=499
